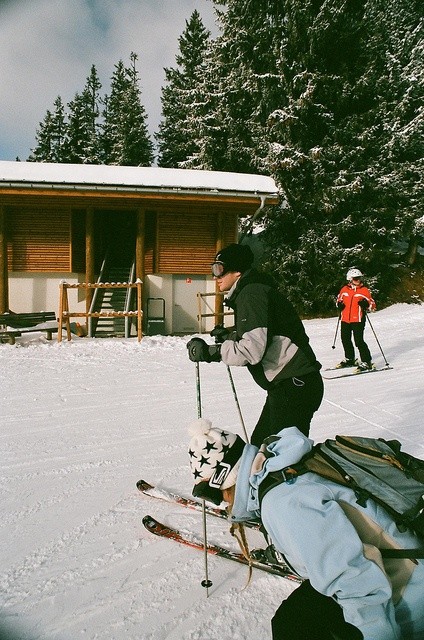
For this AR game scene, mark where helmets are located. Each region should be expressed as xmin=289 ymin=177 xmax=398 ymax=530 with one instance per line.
xmin=346 ymin=268 xmax=364 ymax=280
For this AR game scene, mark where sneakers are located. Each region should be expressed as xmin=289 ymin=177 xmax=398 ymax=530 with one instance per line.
xmin=263 ymin=545 xmax=285 ymax=563
xmin=355 ymin=362 xmax=376 ymax=373
xmin=335 ymin=358 xmax=358 ymax=369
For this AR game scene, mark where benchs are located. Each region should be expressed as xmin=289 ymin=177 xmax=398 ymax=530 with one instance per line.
xmin=0 ymin=312 xmax=58 ymax=345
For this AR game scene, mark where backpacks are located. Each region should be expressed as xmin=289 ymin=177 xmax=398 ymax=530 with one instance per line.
xmin=257 ymin=435 xmax=424 ymax=559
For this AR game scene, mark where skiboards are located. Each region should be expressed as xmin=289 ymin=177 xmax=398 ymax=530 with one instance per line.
xmin=136 ymin=478 xmax=302 ymax=585
xmin=322 ymin=357 xmax=394 ymax=379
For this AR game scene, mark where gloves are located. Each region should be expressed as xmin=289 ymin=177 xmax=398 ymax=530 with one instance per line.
xmin=358 ymin=298 xmax=369 ymax=310
xmin=186 ymin=337 xmax=221 ymax=363
xmin=208 ymin=325 xmax=239 ymax=343
xmin=337 ymin=301 xmax=345 ymax=311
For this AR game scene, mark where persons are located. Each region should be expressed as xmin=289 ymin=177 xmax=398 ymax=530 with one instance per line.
xmin=187 ymin=244 xmax=325 ymax=443
xmin=334 ymin=268 xmax=379 ymax=371
xmin=187 ymin=419 xmax=424 ymax=639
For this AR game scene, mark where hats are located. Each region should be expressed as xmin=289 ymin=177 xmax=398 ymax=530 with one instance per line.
xmin=213 ymin=244 xmax=256 ymax=271
xmin=186 ymin=418 xmax=246 ymax=488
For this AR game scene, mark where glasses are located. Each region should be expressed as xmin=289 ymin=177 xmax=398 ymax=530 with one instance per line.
xmin=210 ymin=263 xmax=229 ymax=277
xmin=353 ymin=276 xmax=363 ymax=280
xmin=193 ymin=435 xmax=244 ymax=504
